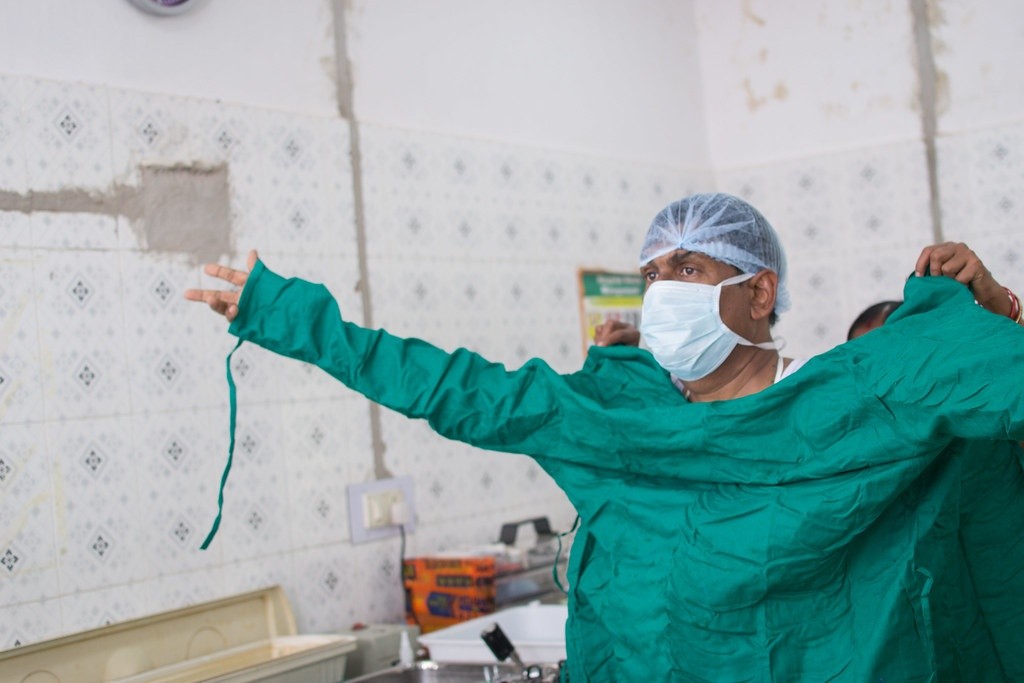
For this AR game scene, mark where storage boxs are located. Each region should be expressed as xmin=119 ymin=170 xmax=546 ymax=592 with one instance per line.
xmin=416 ymin=605 xmax=568 ymax=664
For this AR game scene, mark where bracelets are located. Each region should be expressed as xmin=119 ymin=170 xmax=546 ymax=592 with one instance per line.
xmin=1002 ymin=286 xmax=1023 ymax=324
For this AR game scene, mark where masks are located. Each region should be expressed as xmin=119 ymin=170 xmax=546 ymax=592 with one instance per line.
xmin=636 ymin=272 xmax=785 ymax=383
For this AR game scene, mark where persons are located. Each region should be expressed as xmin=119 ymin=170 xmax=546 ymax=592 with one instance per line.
xmin=594 ymin=242 xmax=1024 ymax=683
xmin=184 ymin=193 xmax=1024 ymax=683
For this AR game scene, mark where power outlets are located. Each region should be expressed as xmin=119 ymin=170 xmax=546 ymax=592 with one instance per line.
xmin=346 ymin=473 xmax=424 ymax=545
